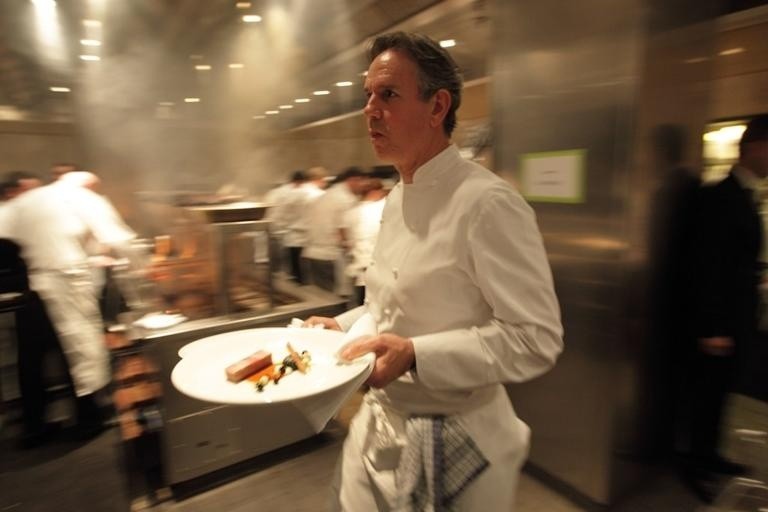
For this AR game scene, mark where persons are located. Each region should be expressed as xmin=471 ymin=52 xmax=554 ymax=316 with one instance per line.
xmin=304 ymin=32 xmax=563 ymax=512
xmin=638 ymin=112 xmax=768 ymax=504
xmin=1 ymin=166 xmax=403 ymax=437
xmin=632 ymin=124 xmax=704 ymax=448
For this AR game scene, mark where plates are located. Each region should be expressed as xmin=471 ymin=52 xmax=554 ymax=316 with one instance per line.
xmin=136 ymin=309 xmax=184 ymax=331
xmin=170 ymin=324 xmax=375 ymax=404
